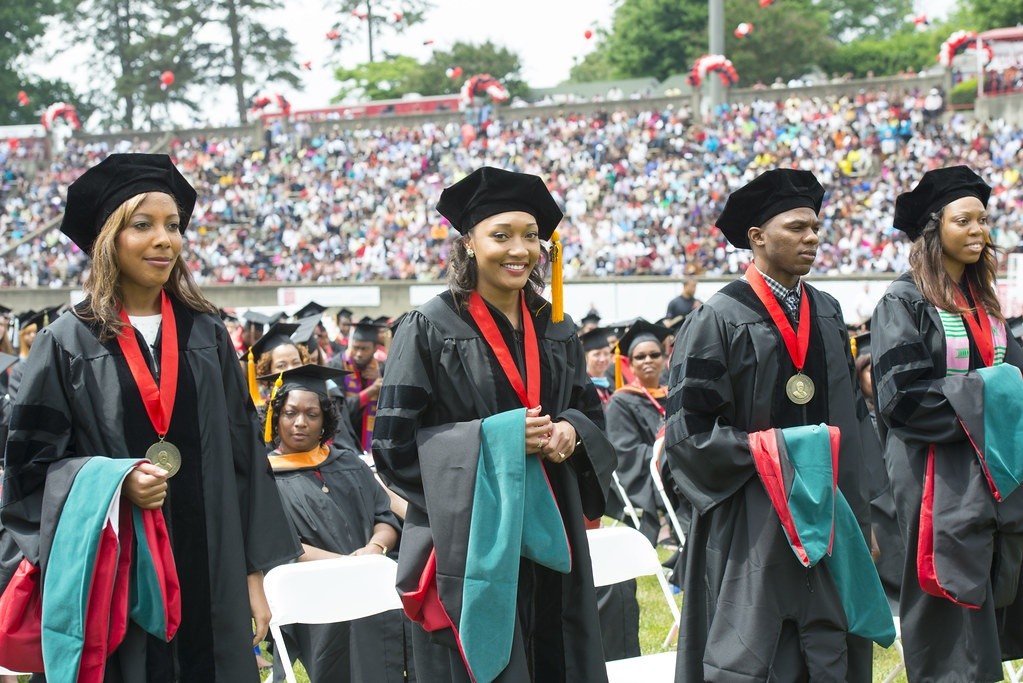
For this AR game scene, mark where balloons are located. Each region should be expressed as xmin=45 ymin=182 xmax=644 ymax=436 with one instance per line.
xmin=734 ymin=22 xmax=752 ymax=37
xmin=759 ymin=0 xmax=776 ymax=7
xmin=326 ymin=10 xmax=434 ymax=45
xmin=585 ymin=30 xmax=591 ymax=39
xmin=915 ymin=16 xmax=928 ymax=25
xmin=161 ymin=71 xmax=174 ymax=85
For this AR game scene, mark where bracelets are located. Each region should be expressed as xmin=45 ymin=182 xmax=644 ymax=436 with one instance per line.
xmin=373 ymin=542 xmax=388 ymax=555
xmin=575 ymin=440 xmax=582 ymax=446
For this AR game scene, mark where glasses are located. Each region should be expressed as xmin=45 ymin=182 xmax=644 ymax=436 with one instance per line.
xmin=630 ymin=351 xmax=660 ymax=361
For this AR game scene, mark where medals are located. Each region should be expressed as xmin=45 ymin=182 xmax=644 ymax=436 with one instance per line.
xmin=145 ymin=440 xmax=181 ymax=479
xmin=785 ymin=373 xmax=815 ymax=405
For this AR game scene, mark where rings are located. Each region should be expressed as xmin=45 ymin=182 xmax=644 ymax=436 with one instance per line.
xmin=538 ymin=440 xmax=543 ymax=447
xmin=559 ymin=452 xmax=565 ymax=458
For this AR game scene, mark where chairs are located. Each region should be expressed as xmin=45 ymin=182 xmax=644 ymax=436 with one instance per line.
xmin=264 ymin=553 xmax=405 ymax=683
xmin=583 ymin=425 xmax=688 ymax=683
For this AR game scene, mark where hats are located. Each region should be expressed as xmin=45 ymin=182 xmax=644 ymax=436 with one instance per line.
xmin=893 ymin=166 xmax=993 ymax=243
xmin=217 ymin=302 xmax=407 ymax=352
xmin=716 ymin=168 xmax=826 ymax=250
xmin=576 ymin=311 xmax=629 ymax=356
xmin=255 ymin=364 xmax=353 ymax=442
xmin=0 ymin=301 xmax=66 ymax=376
xmin=609 ymin=320 xmax=673 ymax=390
xmin=849 ymin=333 xmax=872 ymax=372
xmin=436 ymin=167 xmax=564 ymax=322
xmin=59 ymin=153 xmax=197 ymax=257
xmin=238 ymin=323 xmax=301 ymax=401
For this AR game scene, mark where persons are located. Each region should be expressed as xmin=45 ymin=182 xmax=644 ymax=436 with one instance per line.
xmin=869 ymin=166 xmax=1023 ymax=683
xmin=0 ymin=303 xmax=875 ymax=683
xmin=668 ymin=169 xmax=906 ymax=683
xmin=668 ymin=279 xmax=704 ymax=318
xmin=0 ymin=154 xmax=306 ymax=683
xmin=0 ymin=50 xmax=1022 ymax=279
xmin=372 ymin=167 xmax=618 ymax=683
